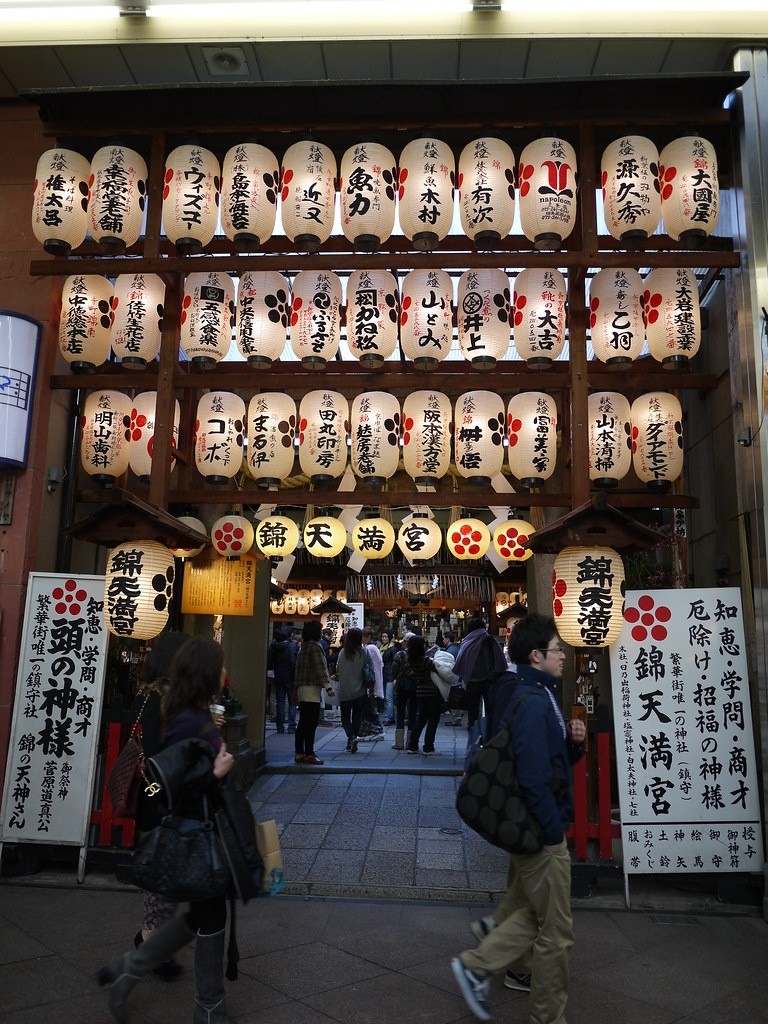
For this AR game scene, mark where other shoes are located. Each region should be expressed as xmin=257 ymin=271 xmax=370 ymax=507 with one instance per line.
xmin=317 ymin=720 xmax=332 ymax=727
xmin=408 ymin=744 xmax=442 ymax=756
xmin=346 ymin=739 xmax=358 ymax=753
xmin=294 ymin=752 xmax=325 ymax=765
xmin=364 ymin=734 xmax=384 ymax=741
xmin=276 ymin=726 xmax=283 ymax=733
xmin=288 ymin=727 xmax=297 ymax=734
xmin=135 ymin=930 xmax=185 ymax=979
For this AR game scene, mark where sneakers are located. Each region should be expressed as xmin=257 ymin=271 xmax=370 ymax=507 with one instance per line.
xmin=453 ymin=956 xmax=492 ymax=1020
xmin=504 ymin=969 xmax=532 ymax=991
xmin=470 ymin=915 xmax=499 ymax=943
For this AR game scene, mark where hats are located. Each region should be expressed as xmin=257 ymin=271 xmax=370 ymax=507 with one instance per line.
xmin=382 ymin=629 xmax=393 ymax=641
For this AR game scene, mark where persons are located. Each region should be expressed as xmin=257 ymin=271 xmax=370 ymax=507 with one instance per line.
xmin=446 ymin=611 xmax=588 ymax=1023
xmin=95 ymin=631 xmax=267 ymax=1024
xmin=265 ymin=616 xmax=508 ymax=765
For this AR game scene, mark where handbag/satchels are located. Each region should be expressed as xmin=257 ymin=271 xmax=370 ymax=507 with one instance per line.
xmin=254 ymin=818 xmax=287 ymax=899
xmin=107 ymin=736 xmax=145 ymax=818
xmin=363 ymin=649 xmax=376 ymax=689
xmin=455 ymin=691 xmax=561 ymax=856
xmin=129 ymin=788 xmax=225 ymax=902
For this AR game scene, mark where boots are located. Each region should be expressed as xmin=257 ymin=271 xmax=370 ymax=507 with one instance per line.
xmin=195 ymin=928 xmax=227 ymax=1024
xmin=407 ymin=730 xmax=414 ymax=749
xmin=96 ymin=912 xmax=195 ymax=1023
xmin=391 ymin=729 xmax=404 ymax=749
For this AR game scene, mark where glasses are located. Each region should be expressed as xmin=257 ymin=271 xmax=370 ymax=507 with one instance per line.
xmin=537 ymin=647 xmax=564 ymax=655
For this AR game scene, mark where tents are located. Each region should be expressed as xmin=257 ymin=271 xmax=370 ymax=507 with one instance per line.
xmin=346 ymin=267 xmax=566 ymax=370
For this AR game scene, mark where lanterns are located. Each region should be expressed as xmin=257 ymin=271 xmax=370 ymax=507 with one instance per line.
xmin=495 ymin=592 xmax=528 ymax=643
xmin=453 ymin=390 xmax=557 ymax=490
xmin=551 ymin=545 xmax=625 ymax=655
xmin=81 ymin=391 xmax=183 ymax=485
xmin=105 ymin=540 xmax=176 ymax=647
xmin=397 ymin=137 xmax=576 ymax=251
xmin=193 ymin=388 xmax=456 ymax=489
xmin=180 ymin=271 xmax=341 ymax=370
xmin=58 ymin=274 xmax=166 ymax=374
xmin=588 ymin=267 xmax=702 ymax=371
xmin=28 ymin=140 xmax=149 ymax=256
xmin=588 ymin=390 xmax=685 ymax=492
xmin=161 ymin=141 xmax=395 ymax=252
xmin=600 ymin=135 xmax=720 ymax=248
xmin=271 ymin=588 xmax=346 ymax=616
xmin=167 ymin=507 xmax=539 ymax=567
xmin=320 ymin=612 xmax=344 ymax=656
